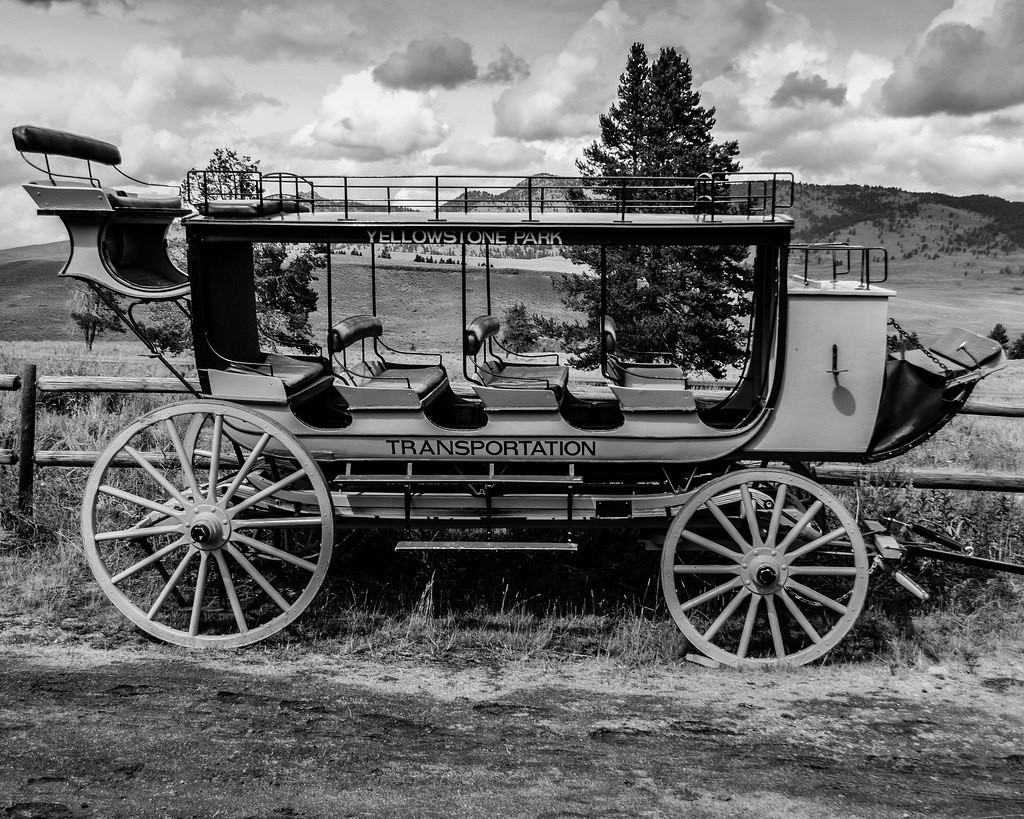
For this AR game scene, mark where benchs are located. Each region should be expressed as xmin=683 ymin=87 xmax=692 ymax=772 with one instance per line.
xmin=328 ymin=313 xmax=450 ymax=411
xmin=202 ymin=318 xmax=336 ymax=407
xmin=599 ymin=314 xmax=698 ymax=413
xmin=11 ymin=124 xmax=193 ymax=223
xmin=462 ymin=315 xmax=570 ymax=411
xmin=184 ymin=168 xmax=316 ymax=223
